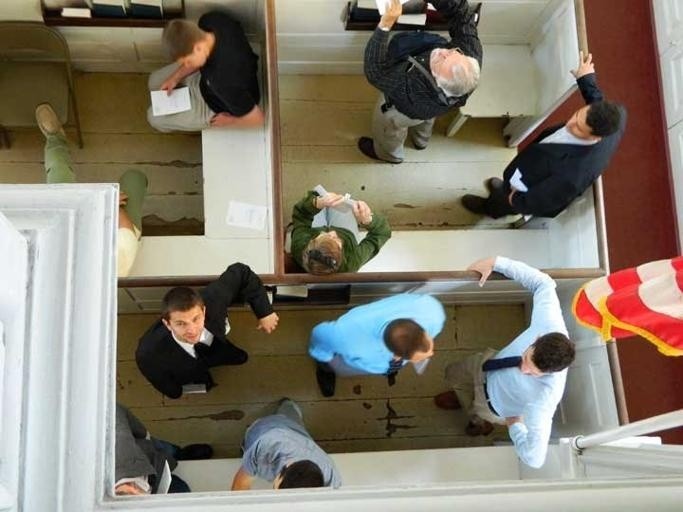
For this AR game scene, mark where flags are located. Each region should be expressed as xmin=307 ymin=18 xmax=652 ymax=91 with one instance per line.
xmin=570 ymin=250 xmax=682 ymax=357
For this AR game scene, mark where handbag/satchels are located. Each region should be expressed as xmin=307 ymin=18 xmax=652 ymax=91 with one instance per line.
xmin=384 ymin=28 xmax=449 ymax=67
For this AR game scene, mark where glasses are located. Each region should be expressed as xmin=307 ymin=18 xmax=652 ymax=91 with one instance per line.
xmin=430 ymin=46 xmax=465 ymax=77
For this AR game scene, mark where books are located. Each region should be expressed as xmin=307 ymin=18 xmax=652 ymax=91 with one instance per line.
xmin=345 ymin=0 xmax=449 ymax=27
xmin=55 ymin=0 xmax=166 ymax=20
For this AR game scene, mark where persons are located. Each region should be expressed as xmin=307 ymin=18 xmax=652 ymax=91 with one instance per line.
xmin=430 ymin=254 xmax=578 ymax=471
xmin=144 ymin=9 xmax=267 ymax=134
xmin=33 ymin=100 xmax=148 ymax=281
xmin=306 ymin=290 xmax=448 ymax=398
xmin=113 ymin=400 xmax=216 ymax=498
xmin=281 ymin=183 xmax=393 ymax=278
xmin=355 ymin=0 xmax=484 ymax=166
xmin=132 ymin=260 xmax=281 ymax=402
xmin=450 ymin=49 xmax=629 ymax=220
xmin=227 ymin=396 xmax=343 ymax=489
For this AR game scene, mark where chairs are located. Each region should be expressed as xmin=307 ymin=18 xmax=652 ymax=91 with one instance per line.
xmin=1 ymin=21 xmax=83 ymax=150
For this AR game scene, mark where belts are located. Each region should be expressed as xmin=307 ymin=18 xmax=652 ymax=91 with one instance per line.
xmin=481 ymin=376 xmax=504 ymax=417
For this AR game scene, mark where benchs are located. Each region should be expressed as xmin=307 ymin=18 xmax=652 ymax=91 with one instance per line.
xmin=283 ymin=229 xmax=581 ymax=272
xmin=118 ymin=233 xmax=275 ymax=277
xmin=187 ymin=42 xmax=268 ymax=236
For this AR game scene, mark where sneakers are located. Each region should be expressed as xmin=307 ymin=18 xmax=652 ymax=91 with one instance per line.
xmin=312 ymin=360 xmax=340 ymax=400
xmin=356 ymin=135 xmax=403 ymax=164
xmin=176 ymin=442 xmax=213 ymax=461
xmin=485 ymin=175 xmax=506 ymax=193
xmin=459 ymin=193 xmax=501 ymax=219
xmin=32 ymin=100 xmax=67 ymax=141
xmin=432 ymin=390 xmax=461 ymax=410
xmin=464 ymin=418 xmax=493 ymax=439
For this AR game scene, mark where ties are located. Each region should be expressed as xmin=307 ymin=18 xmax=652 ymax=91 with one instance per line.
xmin=192 ymin=342 xmax=213 ymax=364
xmin=479 ymin=355 xmax=524 ymax=373
xmin=384 ymin=359 xmax=405 ymax=381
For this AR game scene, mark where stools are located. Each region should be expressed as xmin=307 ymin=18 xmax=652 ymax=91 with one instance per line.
xmin=446 ymin=43 xmax=539 ymax=138
